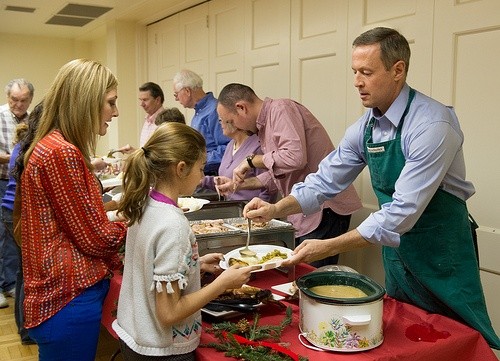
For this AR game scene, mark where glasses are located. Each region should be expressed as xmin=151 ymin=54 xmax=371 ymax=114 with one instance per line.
xmin=173 ymin=87 xmax=184 ymax=97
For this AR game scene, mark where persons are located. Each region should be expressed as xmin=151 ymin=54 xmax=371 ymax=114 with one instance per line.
xmin=243 ymin=27 xmax=500 ymax=351
xmin=214 ymin=82 xmax=363 ymax=267
xmin=12 ymin=56 xmax=128 ymax=361
xmin=138 ymin=81 xmax=165 ymax=154
xmin=2 ymin=118 xmax=38 ymax=345
xmin=194 ymin=112 xmax=277 ymax=203
xmin=173 ymin=70 xmax=232 ymax=176
xmin=119 ymin=107 xmax=186 ymax=156
xmin=110 ymin=121 xmax=263 ymax=360
xmin=0 ymin=77 xmax=34 ymax=307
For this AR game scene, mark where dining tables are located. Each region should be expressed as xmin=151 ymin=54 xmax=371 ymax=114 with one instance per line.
xmin=101 ymin=263 xmax=500 ymax=361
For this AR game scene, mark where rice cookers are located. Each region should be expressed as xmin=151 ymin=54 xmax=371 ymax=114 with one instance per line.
xmin=296 ymin=271 xmax=386 ymax=352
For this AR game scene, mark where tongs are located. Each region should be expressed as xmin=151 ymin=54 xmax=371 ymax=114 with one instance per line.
xmin=203 ymin=298 xmax=258 ymax=313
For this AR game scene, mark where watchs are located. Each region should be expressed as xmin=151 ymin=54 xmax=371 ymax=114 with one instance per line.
xmin=247 ymin=153 xmax=256 ymax=167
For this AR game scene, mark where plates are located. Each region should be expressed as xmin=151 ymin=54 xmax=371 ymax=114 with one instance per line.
xmin=219 ymin=245 xmax=294 ymax=272
xmin=271 ymin=282 xmax=294 ymax=295
xmin=200 ymin=284 xmax=285 ymax=316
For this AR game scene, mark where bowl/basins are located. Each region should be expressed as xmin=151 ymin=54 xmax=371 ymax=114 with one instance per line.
xmin=104 ymin=156 xmax=120 ymax=163
xmin=178 ymin=198 xmax=210 ymax=213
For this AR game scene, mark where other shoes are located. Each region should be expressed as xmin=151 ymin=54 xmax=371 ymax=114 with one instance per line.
xmin=7 ymin=288 xmax=15 ymax=298
xmin=0 ymin=293 xmax=8 ymax=308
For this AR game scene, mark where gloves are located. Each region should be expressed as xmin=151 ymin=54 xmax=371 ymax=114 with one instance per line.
xmin=198 ymin=176 xmax=217 ymax=192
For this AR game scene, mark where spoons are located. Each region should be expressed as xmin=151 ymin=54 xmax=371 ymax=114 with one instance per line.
xmin=239 ymin=210 xmax=257 ymax=257
